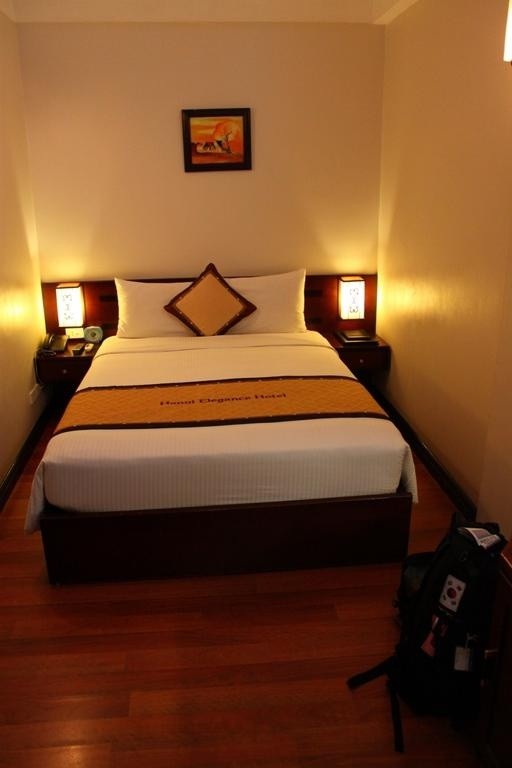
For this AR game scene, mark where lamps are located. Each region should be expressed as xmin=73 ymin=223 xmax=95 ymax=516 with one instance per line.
xmin=56 ymin=283 xmax=85 ymax=327
xmin=339 ymin=277 xmax=366 ymax=320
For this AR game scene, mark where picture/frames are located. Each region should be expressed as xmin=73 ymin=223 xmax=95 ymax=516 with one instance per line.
xmin=180 ymin=108 xmax=252 ymax=172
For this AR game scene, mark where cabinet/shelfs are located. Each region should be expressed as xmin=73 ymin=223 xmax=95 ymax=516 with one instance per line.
xmin=473 ymin=540 xmax=512 ymax=766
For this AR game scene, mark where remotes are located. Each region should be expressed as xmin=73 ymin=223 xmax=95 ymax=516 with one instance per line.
xmin=84 ymin=343 xmax=94 ymax=352
xmin=72 ymin=343 xmax=86 ymax=355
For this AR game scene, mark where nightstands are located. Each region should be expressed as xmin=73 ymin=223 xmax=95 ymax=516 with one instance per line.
xmin=321 ymin=332 xmax=390 ymax=391
xmin=33 ymin=337 xmax=101 ymax=404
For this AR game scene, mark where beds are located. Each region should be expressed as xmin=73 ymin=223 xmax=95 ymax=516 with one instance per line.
xmin=24 ymin=331 xmax=419 ymax=585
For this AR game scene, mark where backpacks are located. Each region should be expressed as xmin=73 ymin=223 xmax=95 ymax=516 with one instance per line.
xmin=392 ymin=518 xmax=512 ymax=724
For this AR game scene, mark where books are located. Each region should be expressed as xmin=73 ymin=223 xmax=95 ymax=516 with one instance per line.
xmin=342 ymin=328 xmax=372 ymax=340
xmin=333 ymin=333 xmax=379 ymax=347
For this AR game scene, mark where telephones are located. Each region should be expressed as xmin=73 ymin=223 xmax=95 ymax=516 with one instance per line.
xmin=42 ymin=333 xmax=68 ymax=352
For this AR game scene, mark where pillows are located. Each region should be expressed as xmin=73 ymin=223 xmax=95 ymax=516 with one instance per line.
xmin=164 ymin=262 xmax=257 ymax=338
xmin=219 ymin=269 xmax=308 ymax=334
xmin=112 ymin=277 xmax=194 ymax=338
